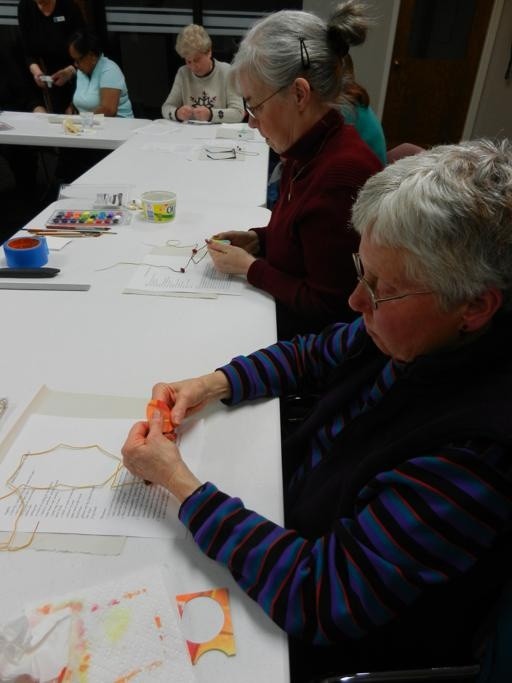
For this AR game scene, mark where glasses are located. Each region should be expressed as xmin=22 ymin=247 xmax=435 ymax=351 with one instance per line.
xmin=76 ymin=54 xmax=87 ymax=64
xmin=351 ymin=251 xmax=434 ymax=311
xmin=245 ymin=82 xmax=295 ymax=120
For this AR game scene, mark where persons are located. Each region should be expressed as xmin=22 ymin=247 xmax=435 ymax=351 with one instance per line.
xmin=121 ymin=134 xmax=512 ymax=683
xmin=64 ymin=31 xmax=135 ymax=118
xmin=325 ymin=49 xmax=387 ymax=170
xmin=12 ymin=0 xmax=87 ymax=114
xmin=161 ymin=23 xmax=245 ymax=123
xmin=203 ymin=0 xmax=385 ymax=338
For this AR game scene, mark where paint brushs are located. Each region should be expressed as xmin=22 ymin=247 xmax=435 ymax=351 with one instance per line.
xmin=18 ymin=226 xmax=117 ymax=238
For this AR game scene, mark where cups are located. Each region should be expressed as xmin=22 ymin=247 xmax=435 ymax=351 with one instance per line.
xmin=81 ymin=112 xmax=94 ymax=128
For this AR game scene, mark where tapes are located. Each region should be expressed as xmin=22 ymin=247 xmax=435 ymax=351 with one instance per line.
xmin=3 ymin=236 xmax=49 ymax=268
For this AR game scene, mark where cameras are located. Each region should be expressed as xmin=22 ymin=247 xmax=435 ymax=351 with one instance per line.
xmin=40 ymin=76 xmax=53 ymax=88
